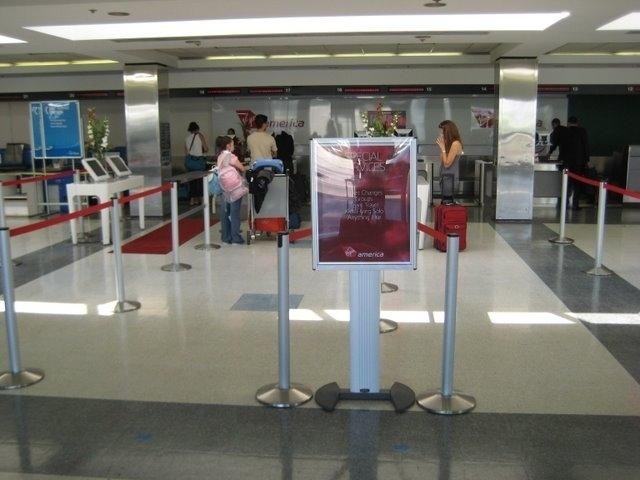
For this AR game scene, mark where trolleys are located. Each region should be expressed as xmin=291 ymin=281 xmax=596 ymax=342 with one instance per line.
xmin=247 ymin=160 xmax=290 ymax=245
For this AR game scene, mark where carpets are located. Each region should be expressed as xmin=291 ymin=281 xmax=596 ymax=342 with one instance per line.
xmin=108 ymin=215 xmax=220 ymax=254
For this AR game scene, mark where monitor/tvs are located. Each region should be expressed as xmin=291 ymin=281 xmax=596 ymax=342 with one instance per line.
xmin=218 ymin=135 xmax=235 ymax=139
xmin=394 ymin=129 xmax=413 ymax=137
xmin=105 ymin=155 xmax=133 ymax=178
xmin=81 ymin=158 xmax=111 ymax=182
xmin=354 ymin=131 xmax=371 ymax=138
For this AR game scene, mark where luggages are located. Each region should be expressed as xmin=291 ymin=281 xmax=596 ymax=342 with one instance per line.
xmin=434 ymin=173 xmax=467 ymax=252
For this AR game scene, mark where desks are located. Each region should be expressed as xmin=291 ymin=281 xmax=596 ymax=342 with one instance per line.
xmin=65 ymin=174 xmax=145 ymax=246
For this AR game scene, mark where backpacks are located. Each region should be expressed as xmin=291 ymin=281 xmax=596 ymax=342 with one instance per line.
xmin=207 ymin=153 xmax=249 ymax=203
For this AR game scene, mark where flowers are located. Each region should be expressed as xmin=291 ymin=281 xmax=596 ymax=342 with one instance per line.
xmin=361 ymin=98 xmax=402 ymax=138
xmin=85 ymin=106 xmax=113 ymax=160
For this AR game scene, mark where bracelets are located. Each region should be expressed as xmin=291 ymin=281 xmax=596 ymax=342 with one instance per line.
xmin=443 ymin=151 xmax=446 ymax=153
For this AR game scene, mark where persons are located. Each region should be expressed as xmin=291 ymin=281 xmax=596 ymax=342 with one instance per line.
xmin=185 ymin=122 xmax=208 ymax=205
xmin=436 ymin=120 xmax=463 ymax=204
xmin=546 ymin=116 xmax=590 ymax=210
xmin=215 ymin=129 xmax=245 ymax=245
xmin=275 ymin=131 xmax=294 ymax=173
xmin=246 ymin=115 xmax=278 ymax=170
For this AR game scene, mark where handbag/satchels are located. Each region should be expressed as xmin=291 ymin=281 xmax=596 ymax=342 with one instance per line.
xmin=253 ymin=160 xmax=284 ymax=174
